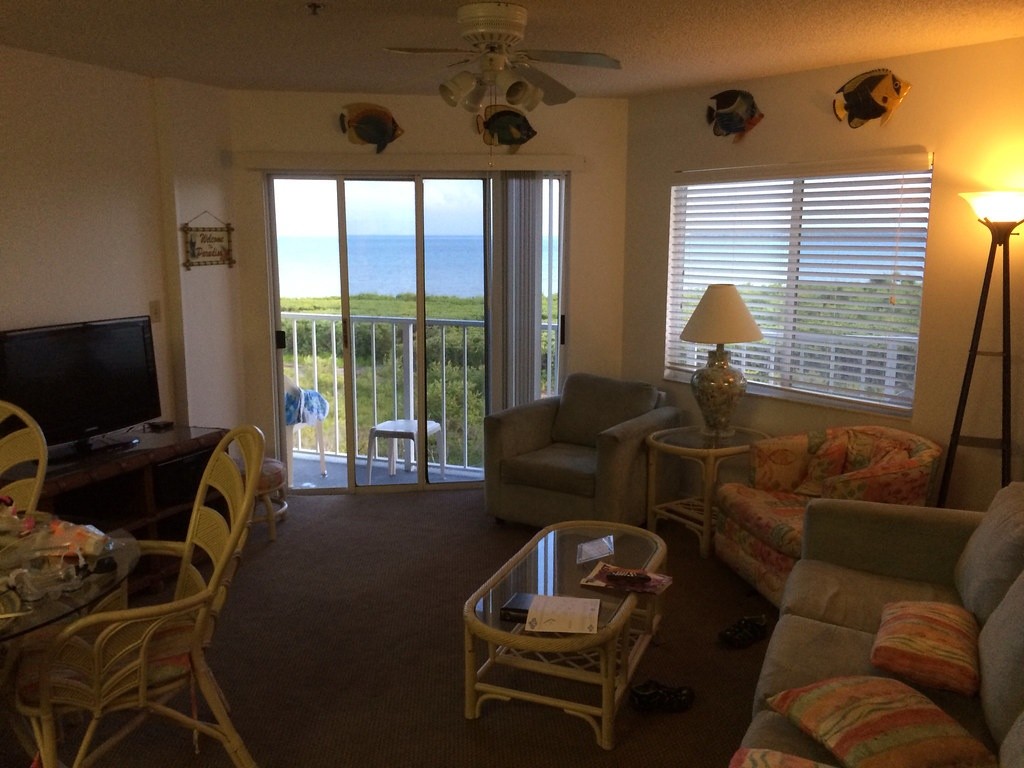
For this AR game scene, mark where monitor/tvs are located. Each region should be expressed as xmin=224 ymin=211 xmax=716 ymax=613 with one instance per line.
xmin=0 ymin=315 xmax=162 ymax=466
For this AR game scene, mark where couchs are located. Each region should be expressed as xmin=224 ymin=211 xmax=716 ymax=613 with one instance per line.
xmin=714 ymin=424 xmax=942 ymax=610
xmin=483 ymin=372 xmax=681 ymax=530
xmin=739 ymin=481 xmax=1024 ymax=768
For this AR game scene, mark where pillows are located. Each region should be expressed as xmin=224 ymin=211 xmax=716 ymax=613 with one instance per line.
xmin=729 ymin=748 xmax=836 ymax=768
xmin=794 ymin=430 xmax=911 ymax=496
xmin=764 ymin=675 xmax=1000 ymax=768
xmin=870 ymin=601 xmax=980 ymax=693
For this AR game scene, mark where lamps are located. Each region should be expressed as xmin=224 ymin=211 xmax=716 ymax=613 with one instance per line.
xmin=681 ymin=284 xmax=761 ymax=439
xmin=438 ymin=53 xmax=545 ymax=167
xmin=938 ymin=191 xmax=1023 ymax=509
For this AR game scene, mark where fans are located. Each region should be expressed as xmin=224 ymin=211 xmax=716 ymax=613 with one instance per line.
xmin=382 ymin=3 xmax=623 ymax=167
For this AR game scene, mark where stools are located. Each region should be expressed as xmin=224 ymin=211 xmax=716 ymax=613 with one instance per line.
xmin=231 ymin=456 xmax=289 ymax=541
xmin=366 ymin=420 xmax=446 ymax=485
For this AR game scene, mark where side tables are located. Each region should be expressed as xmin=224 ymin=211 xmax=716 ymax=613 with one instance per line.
xmin=645 ymin=425 xmax=773 ymax=560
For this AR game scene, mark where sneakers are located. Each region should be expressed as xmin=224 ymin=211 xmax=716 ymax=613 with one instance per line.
xmin=719 ymin=613 xmax=769 ymax=647
xmin=622 ymin=678 xmax=695 ymax=713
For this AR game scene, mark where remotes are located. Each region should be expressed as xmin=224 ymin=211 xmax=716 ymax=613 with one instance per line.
xmin=606 ymin=571 xmax=651 ymax=584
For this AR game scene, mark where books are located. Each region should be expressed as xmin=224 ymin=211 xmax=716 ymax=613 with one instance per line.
xmin=580 ymin=561 xmax=673 ymax=596
xmin=500 ymin=592 xmax=536 ymax=624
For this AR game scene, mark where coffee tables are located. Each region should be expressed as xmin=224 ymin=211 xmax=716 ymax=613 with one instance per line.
xmin=463 ymin=520 xmax=669 ymax=751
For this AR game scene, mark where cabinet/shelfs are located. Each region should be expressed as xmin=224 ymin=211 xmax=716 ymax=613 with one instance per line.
xmin=1 ymin=426 xmax=234 ymax=596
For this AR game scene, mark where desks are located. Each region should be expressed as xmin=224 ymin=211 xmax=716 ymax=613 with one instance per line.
xmin=0 ymin=514 xmax=142 ymax=760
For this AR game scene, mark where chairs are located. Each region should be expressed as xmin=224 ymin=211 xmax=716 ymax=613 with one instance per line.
xmin=283 ymin=374 xmax=330 ymax=488
xmin=0 ymin=401 xmax=268 ymax=768
